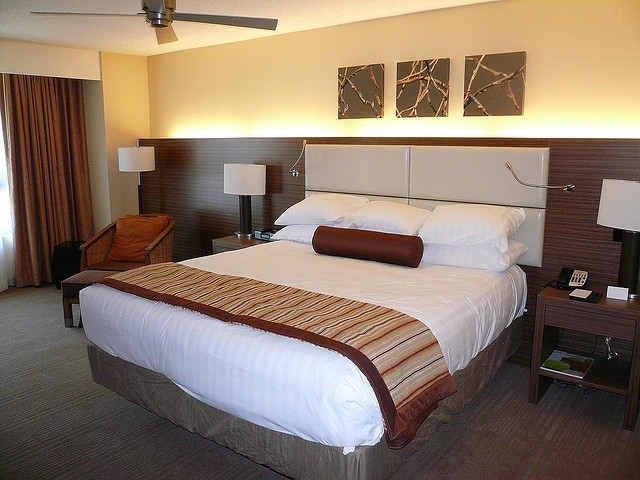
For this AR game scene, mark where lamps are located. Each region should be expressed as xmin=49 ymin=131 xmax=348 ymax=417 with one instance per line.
xmin=223 ymin=163 xmax=267 ymax=238
xmin=118 ymin=147 xmax=155 ymax=213
xmin=596 ymin=178 xmax=640 ymax=300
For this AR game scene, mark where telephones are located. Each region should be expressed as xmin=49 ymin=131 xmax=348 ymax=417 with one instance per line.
xmin=545 ymin=267 xmax=589 ymax=290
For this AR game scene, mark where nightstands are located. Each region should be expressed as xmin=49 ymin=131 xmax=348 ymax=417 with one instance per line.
xmin=528 ymin=285 xmax=638 ymax=432
xmin=211 ymin=232 xmax=271 ymax=255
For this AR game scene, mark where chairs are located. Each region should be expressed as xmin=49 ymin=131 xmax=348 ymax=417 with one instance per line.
xmin=61 ymin=213 xmax=177 ymax=328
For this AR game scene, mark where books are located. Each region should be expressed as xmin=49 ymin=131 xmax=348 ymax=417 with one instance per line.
xmin=539 ymin=348 xmax=595 ymax=378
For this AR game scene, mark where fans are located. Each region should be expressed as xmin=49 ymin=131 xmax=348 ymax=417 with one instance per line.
xmin=30 ymin=0 xmax=278 ymax=44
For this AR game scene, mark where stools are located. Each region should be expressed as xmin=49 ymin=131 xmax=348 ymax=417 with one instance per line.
xmin=59 ymin=271 xmax=124 ymax=327
xmin=52 ymin=241 xmax=85 ymax=290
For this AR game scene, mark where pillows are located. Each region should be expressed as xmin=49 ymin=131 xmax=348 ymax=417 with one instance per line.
xmin=269 ymin=225 xmax=335 ymax=244
xmin=103 ymin=215 xmax=169 ymax=262
xmin=332 ymin=200 xmax=432 ymax=236
xmin=421 ymin=239 xmax=529 ymax=272
xmin=419 ymin=204 xmax=527 ymax=253
xmin=273 ymin=191 xmax=370 ymax=225
xmin=312 ymin=226 xmax=424 ymax=268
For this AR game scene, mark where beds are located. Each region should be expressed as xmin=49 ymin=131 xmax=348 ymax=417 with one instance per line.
xmin=77 ymin=145 xmax=553 ymax=480
